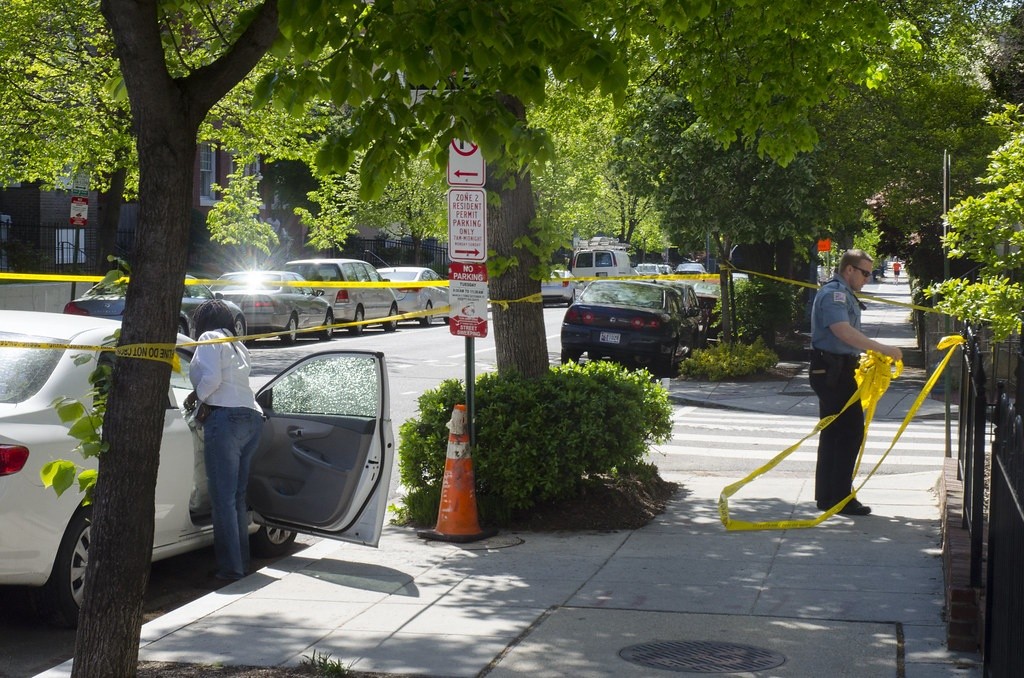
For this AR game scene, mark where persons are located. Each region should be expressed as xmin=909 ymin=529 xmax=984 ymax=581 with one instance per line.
xmin=190 ymin=299 xmax=262 ymax=588
xmin=893 ymin=258 xmax=901 ymax=285
xmin=809 ymin=250 xmax=902 ymax=515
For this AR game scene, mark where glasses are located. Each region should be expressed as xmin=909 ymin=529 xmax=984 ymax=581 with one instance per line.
xmin=852 ymin=264 xmax=872 ymax=278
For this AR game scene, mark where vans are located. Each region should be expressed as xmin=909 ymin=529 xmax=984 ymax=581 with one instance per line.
xmin=284 ymin=259 xmax=398 ymax=335
xmin=571 ymin=236 xmax=638 ymax=278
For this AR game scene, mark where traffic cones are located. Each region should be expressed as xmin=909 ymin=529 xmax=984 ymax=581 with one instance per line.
xmin=415 ymin=405 xmax=500 ymax=541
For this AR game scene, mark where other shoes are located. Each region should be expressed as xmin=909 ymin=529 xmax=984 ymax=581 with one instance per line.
xmin=817 ymin=496 xmax=871 ymax=516
xmin=193 ymin=566 xmax=225 ymax=590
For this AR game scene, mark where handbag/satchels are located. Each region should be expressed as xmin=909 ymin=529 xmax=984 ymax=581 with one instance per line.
xmin=183 ymin=389 xmax=212 ymax=430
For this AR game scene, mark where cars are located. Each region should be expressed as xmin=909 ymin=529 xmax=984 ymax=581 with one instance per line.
xmin=638 ymin=279 xmax=710 ymax=348
xmin=541 ymin=269 xmax=585 ymax=307
xmin=63 ymin=275 xmax=247 ymax=345
xmin=0 ymin=310 xmax=395 ymax=626
xmin=634 ymin=264 xmax=659 ymax=276
xmin=197 ymin=271 xmax=335 ymax=346
xmin=560 ymin=279 xmax=699 ymax=377
xmin=655 ymin=265 xmax=673 ymax=275
xmin=687 ymin=272 xmax=750 ymax=333
xmin=673 ymin=263 xmax=706 ymax=274
xmin=358 ymin=267 xmax=449 ymax=327
